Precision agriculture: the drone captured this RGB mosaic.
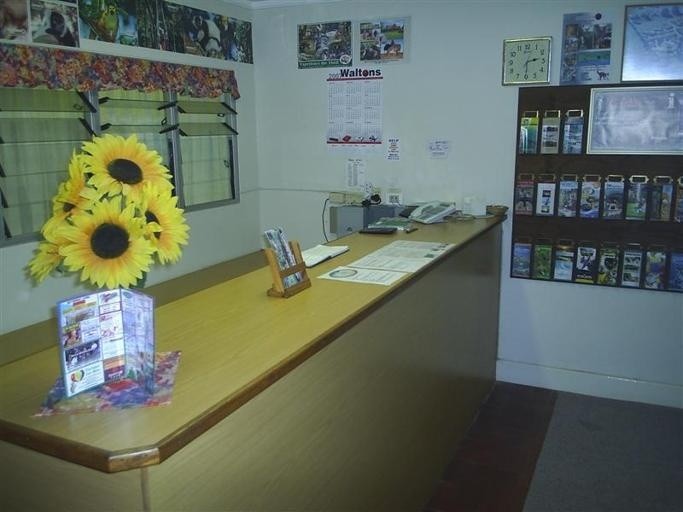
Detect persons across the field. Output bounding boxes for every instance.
[64,330,81,346]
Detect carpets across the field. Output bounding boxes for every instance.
[428,382,558,511]
[521,390,683,512]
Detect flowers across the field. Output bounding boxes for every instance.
[22,132,190,292]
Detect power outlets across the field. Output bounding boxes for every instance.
[329,192,345,203]
[389,195,399,203]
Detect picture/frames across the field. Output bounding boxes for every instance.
[586,87,683,156]
[620,2,683,84]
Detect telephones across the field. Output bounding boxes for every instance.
[408,201,457,225]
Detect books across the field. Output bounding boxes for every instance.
[367,217,409,230]
[301,242,349,268]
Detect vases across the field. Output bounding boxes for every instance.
[118,271,147,290]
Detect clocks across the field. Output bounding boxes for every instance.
[502,36,552,86]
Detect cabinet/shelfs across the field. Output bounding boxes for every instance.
[496,84,683,409]
